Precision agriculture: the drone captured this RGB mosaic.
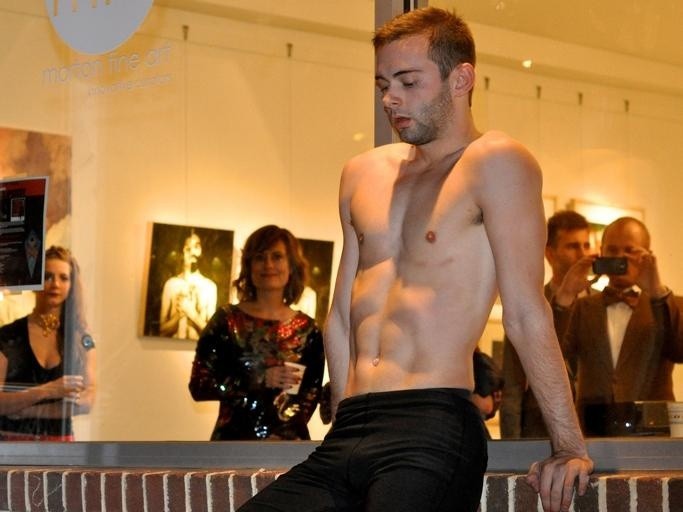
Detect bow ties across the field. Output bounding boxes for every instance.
[602,286,640,310]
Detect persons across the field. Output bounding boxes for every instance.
[158,233,218,341]
[468,348,504,419]
[230,4,594,512]
[0,246,95,441]
[549,216,683,434]
[285,284,318,320]
[500,210,604,440]
[187,224,326,442]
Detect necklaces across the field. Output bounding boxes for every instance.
[31,307,61,337]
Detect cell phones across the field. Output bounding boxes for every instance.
[593,257,627,274]
